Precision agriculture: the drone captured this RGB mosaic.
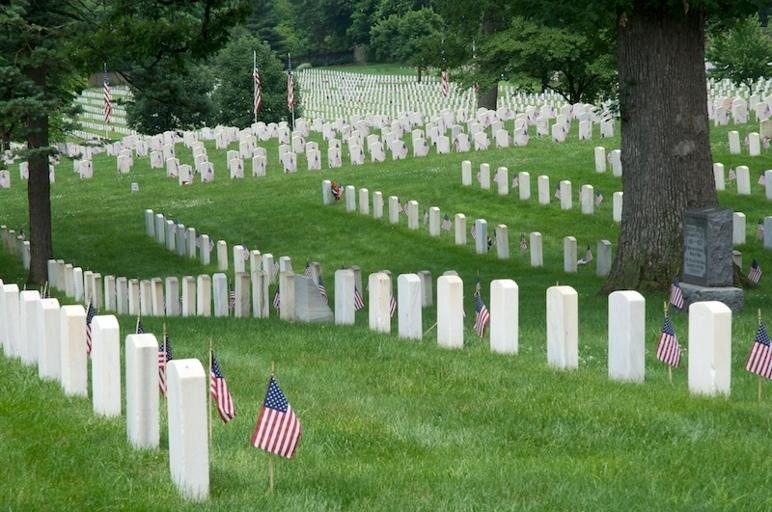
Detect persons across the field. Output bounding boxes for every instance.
[397,194,594,265]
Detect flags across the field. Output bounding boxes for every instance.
[438,40,451,97]
[207,346,238,424]
[21,280,174,401]
[655,258,772,381]
[250,51,263,115]
[224,253,491,341]
[248,374,303,460]
[101,60,115,126]
[727,127,772,251]
[282,50,298,116]
[475,169,604,208]
[331,185,344,200]
